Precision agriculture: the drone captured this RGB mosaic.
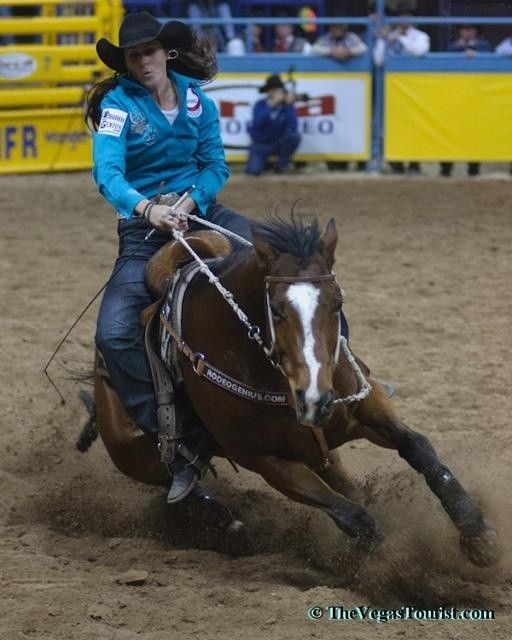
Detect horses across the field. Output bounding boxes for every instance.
[56,193,502,570]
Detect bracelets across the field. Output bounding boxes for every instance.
[146,202,156,225]
[139,201,153,220]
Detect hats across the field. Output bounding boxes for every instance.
[96,12,192,73]
[258,75,285,92]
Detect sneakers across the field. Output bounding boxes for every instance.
[376,384,393,399]
[168,447,211,503]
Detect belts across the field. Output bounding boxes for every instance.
[116,192,181,219]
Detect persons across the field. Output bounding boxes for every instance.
[244,9,512,178]
[84,11,275,503]
